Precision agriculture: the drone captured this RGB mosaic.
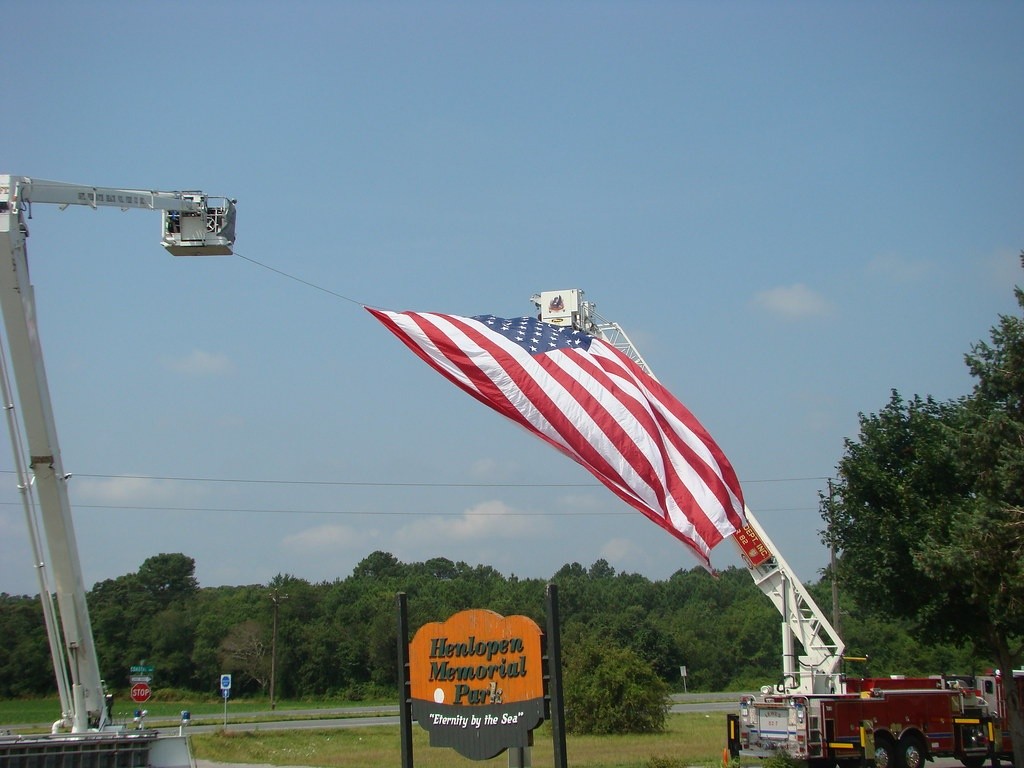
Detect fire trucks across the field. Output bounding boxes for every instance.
[527,287,1024,768]
[1,173,235,768]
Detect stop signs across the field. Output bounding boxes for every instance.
[130,683,151,703]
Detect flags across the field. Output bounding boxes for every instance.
[364,306,748,577]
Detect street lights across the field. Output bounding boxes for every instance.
[268,588,290,711]
